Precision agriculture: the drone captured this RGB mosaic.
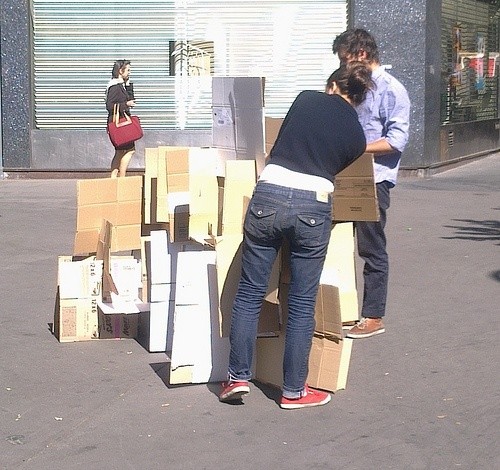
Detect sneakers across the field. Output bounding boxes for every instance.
[220,381,250,401]
[280,385,331,408]
[347,317,385,339]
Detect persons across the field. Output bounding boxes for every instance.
[104,60,136,179]
[220,61,375,410]
[333,29,410,339]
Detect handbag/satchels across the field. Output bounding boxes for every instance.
[108,103,143,148]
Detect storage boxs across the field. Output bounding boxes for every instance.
[54,77,380,392]
